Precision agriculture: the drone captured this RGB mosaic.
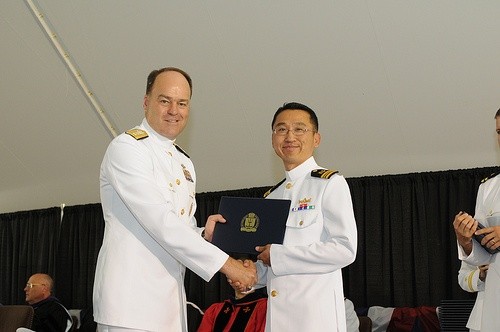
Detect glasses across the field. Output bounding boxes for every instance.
[272,126,316,136]
[26,282,49,288]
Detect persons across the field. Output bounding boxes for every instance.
[197,256,361,332]
[453,108,500,332]
[227,103,357,332]
[93,66,258,332]
[24,273,76,332]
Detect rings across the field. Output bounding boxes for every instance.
[247,284,252,291]
